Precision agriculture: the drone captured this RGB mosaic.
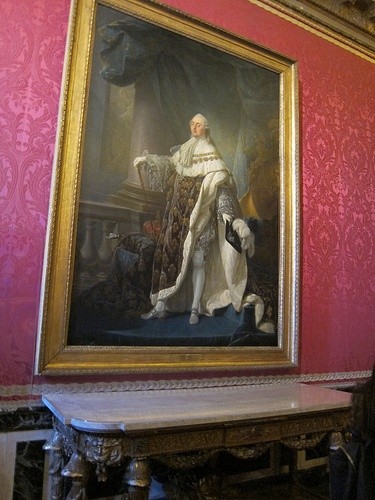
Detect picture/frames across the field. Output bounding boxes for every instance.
[33,0,301,375]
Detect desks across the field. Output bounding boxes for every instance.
[41,382,366,500]
